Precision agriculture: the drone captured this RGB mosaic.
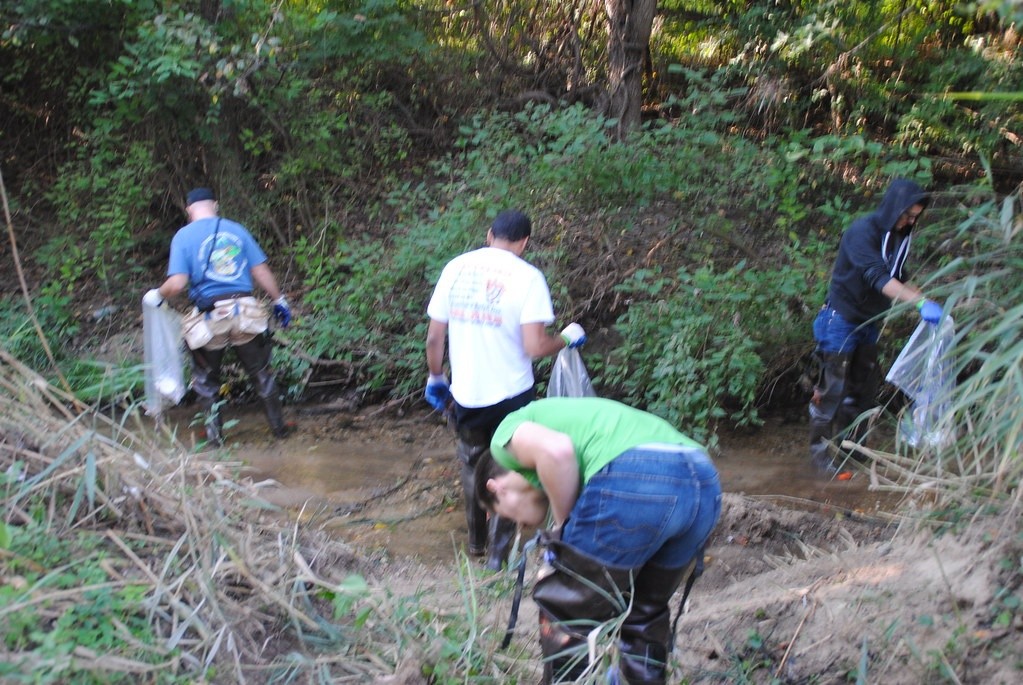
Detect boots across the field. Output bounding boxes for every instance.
[531,529,644,685]
[457,439,490,554]
[487,514,516,573]
[230,332,298,439]
[193,347,225,446]
[609,565,684,685]
[838,367,885,469]
[805,349,853,481]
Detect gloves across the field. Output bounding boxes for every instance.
[561,322,587,346]
[144,288,165,309]
[916,297,943,321]
[424,374,450,410]
[272,298,291,325]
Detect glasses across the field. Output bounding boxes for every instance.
[906,209,919,219]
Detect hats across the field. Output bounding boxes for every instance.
[186,188,215,204]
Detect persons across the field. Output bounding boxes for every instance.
[809,179,942,476]
[475,397,723,685]
[143,187,291,447]
[425,211,586,588]
